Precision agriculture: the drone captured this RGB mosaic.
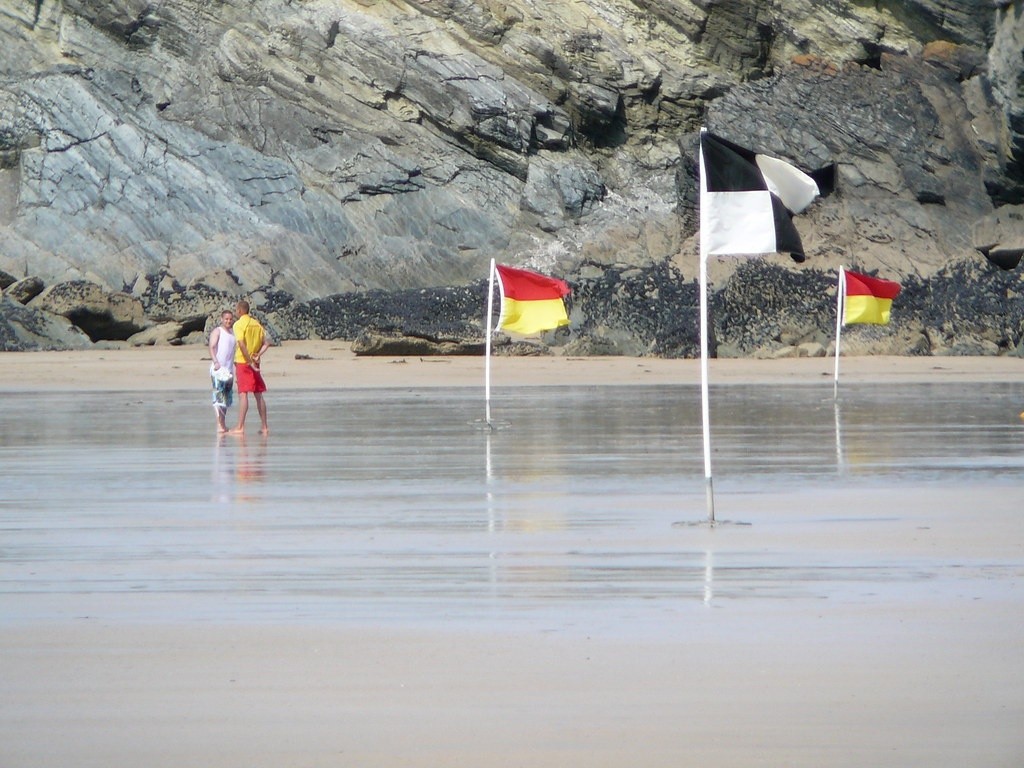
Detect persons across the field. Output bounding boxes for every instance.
[209,311,238,433]
[228,302,271,436]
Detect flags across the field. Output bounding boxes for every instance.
[494,265,573,339]
[702,129,820,261]
[840,267,902,327]
